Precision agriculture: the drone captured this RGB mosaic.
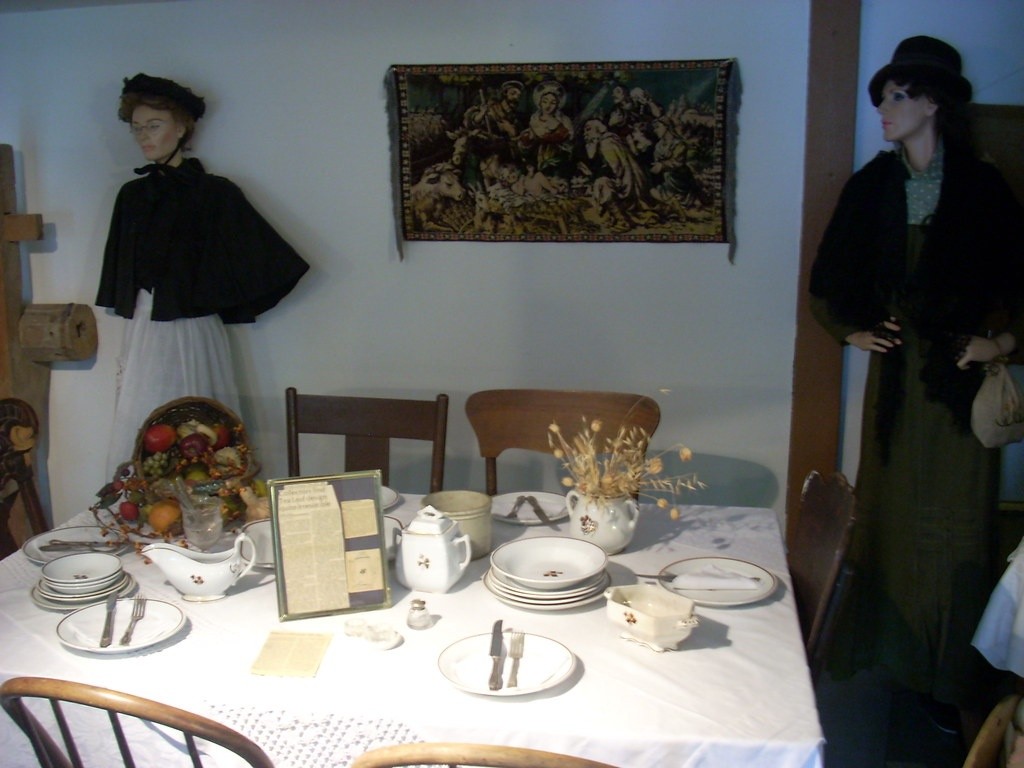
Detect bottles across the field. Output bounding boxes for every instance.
[407,600,432,630]
[391,506,471,593]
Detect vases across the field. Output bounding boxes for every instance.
[566,490,637,557]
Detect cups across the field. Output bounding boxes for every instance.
[181,496,223,551]
[420,489,493,561]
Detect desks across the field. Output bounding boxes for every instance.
[0,494,825,768]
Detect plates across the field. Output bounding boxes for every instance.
[381,485,398,510]
[32,552,135,610]
[438,632,576,696]
[484,537,615,611]
[656,557,777,606]
[23,524,130,563]
[492,491,569,524]
[238,518,276,569]
[55,597,186,654]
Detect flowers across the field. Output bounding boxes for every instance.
[547,385,708,521]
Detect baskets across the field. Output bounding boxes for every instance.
[132,396,252,490]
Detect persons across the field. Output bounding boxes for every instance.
[807,35,1024,733]
[95,73,311,483]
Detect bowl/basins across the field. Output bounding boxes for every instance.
[604,585,699,652]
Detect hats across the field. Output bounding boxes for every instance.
[868,34,973,116]
[124,72,206,122]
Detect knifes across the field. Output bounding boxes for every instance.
[488,620,503,690]
[630,572,675,582]
[100,590,118,647]
[526,496,560,531]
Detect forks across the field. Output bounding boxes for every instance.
[120,595,146,645]
[509,632,524,687]
[504,496,525,519]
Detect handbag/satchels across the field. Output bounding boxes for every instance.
[970,360,1024,448]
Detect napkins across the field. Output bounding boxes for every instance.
[670,565,763,590]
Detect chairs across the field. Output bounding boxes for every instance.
[0,388,862,768]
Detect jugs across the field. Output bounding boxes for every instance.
[141,534,257,601]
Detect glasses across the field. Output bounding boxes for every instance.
[127,118,178,137]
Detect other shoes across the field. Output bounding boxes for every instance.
[936,698,960,734]
